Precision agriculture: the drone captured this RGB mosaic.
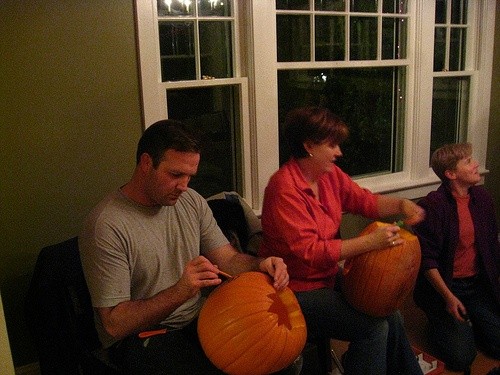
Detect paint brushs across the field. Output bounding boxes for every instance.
[218,270,233,279]
[458,308,472,327]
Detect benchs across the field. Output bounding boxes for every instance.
[27,191,330,375]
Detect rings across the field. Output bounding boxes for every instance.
[392,241,396,247]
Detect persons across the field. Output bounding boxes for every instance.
[259,107,428,375]
[410,143,500,373]
[77,118,307,375]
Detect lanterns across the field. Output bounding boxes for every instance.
[196,272,307,375]
[340,221,422,319]
